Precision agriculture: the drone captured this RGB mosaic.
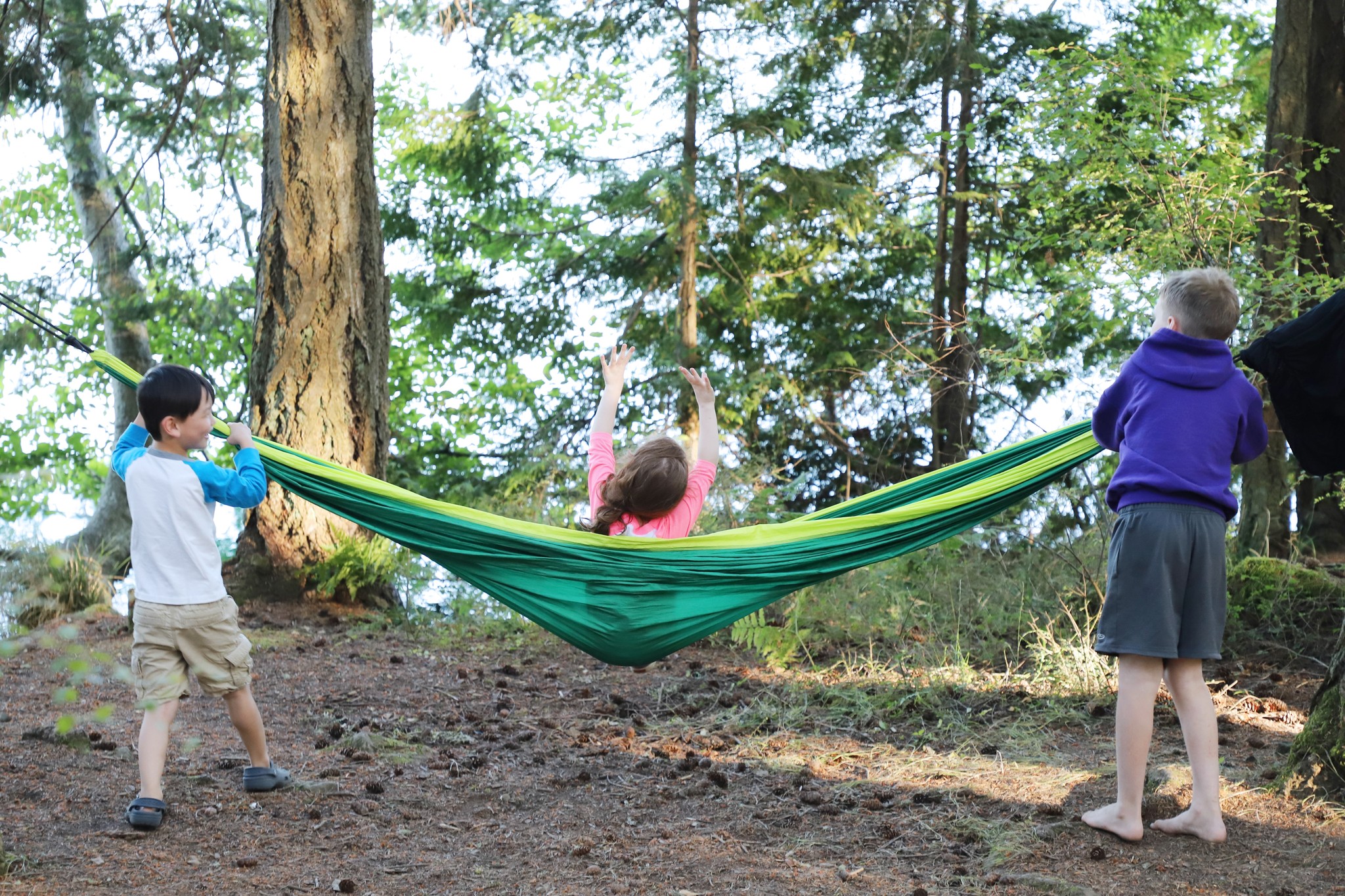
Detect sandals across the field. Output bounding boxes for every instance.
[242,763,292,790]
[125,798,165,827]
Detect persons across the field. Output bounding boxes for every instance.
[580,344,720,672]
[1080,265,1270,840]
[110,364,292,827]
